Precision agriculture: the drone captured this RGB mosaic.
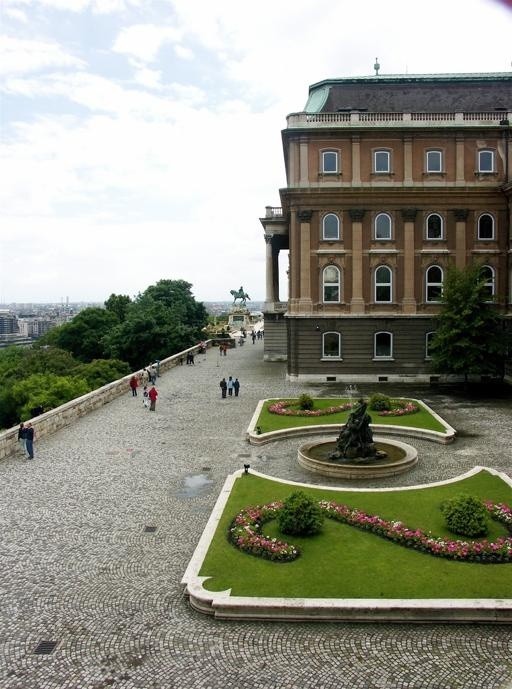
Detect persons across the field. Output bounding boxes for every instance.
[186,351,190,364]
[219,378,227,398]
[149,386,158,411]
[228,376,234,397]
[24,423,34,460]
[136,362,158,387]
[130,376,138,397]
[238,285,243,294]
[234,378,240,397]
[243,329,264,344]
[224,344,226,356]
[219,345,224,356]
[335,398,372,459]
[18,422,28,455]
[143,386,149,408]
[190,352,194,364]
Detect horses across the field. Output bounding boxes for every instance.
[230,290,250,304]
